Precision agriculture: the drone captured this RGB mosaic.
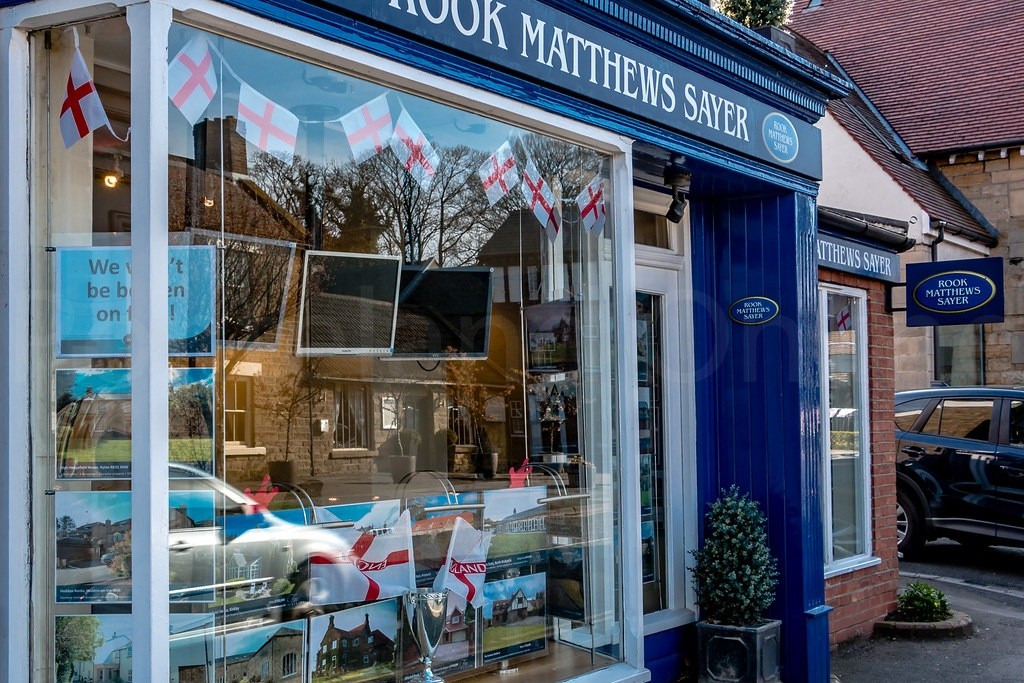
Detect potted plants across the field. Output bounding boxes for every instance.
[686,484,782,683]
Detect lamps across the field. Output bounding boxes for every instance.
[205,177,217,207]
[105,156,125,187]
[663,163,691,224]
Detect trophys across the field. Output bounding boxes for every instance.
[402,587,448,682]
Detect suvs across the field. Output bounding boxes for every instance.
[895,385,1024,565]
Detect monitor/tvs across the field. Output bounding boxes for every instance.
[184,225,494,362]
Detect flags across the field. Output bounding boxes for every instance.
[310,508,417,606]
[58,48,108,149]
[440,516,492,610]
[574,173,606,237]
[235,82,300,167]
[339,93,440,191]
[478,141,561,244]
[167,32,218,128]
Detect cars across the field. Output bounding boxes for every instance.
[53,460,361,680]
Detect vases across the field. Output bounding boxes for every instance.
[266,460,297,492]
[471,452,499,480]
[388,455,416,485]
[563,462,595,488]
